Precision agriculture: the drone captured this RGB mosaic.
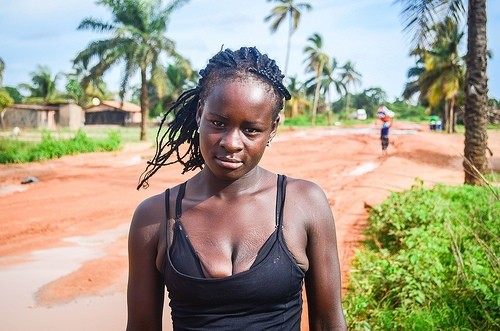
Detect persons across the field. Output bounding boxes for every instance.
[126,46,347,330]
[380,118,392,149]
